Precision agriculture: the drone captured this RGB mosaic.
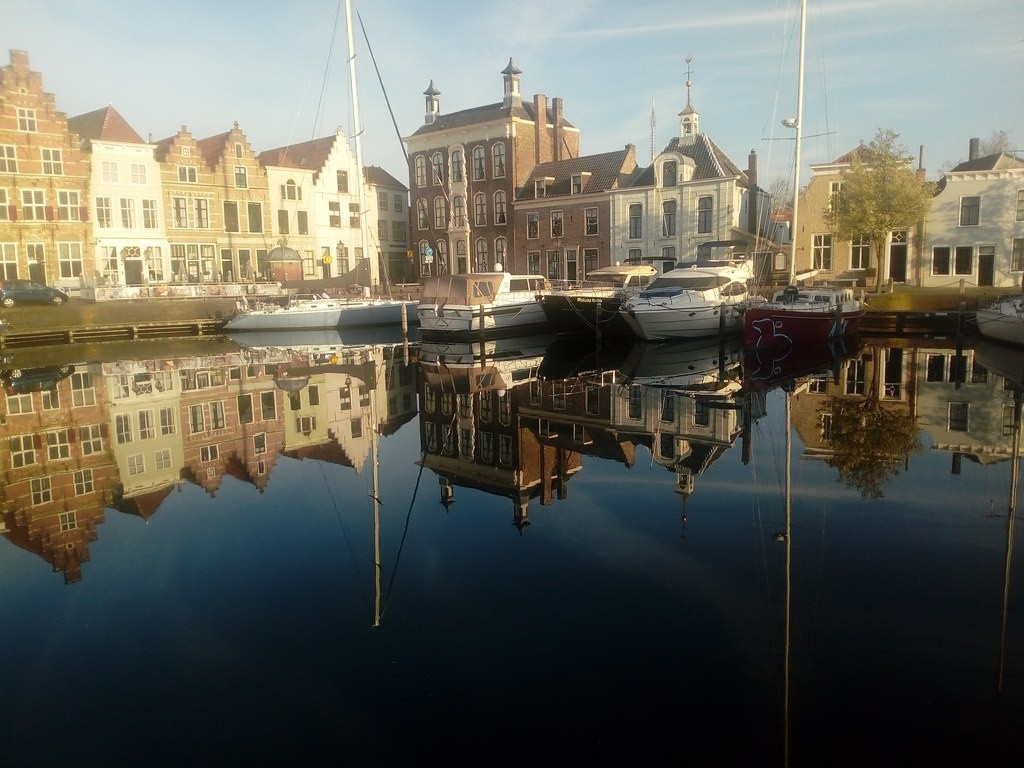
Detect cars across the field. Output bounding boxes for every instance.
[0,280,70,308]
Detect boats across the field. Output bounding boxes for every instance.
[616,340,745,399]
[619,259,748,339]
[416,273,551,340]
[412,341,548,397]
[225,332,437,629]
[536,266,656,331]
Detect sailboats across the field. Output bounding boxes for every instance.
[976,293,1024,345]
[972,346,1023,695]
[225,1,419,332]
[743,1,862,342]
[741,341,857,767]
